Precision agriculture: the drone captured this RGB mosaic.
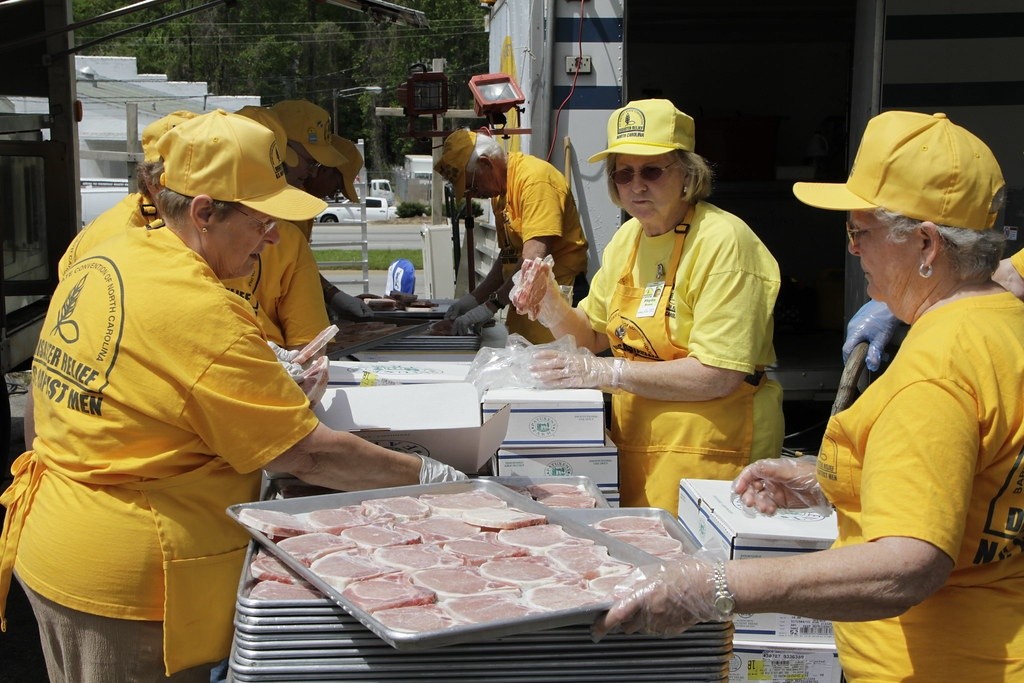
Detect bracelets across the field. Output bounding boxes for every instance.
[611,358,625,392]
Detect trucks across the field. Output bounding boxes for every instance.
[336,178,395,207]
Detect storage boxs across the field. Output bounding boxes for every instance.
[264,383,620,509]
[698,116,791,180]
[677,478,842,683]
[816,267,844,330]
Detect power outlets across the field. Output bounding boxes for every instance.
[566,56,592,74]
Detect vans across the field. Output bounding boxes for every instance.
[315,196,388,225]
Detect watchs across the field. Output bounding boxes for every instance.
[489,292,505,310]
[713,561,735,618]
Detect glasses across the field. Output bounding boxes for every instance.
[287,143,322,170]
[462,155,488,197]
[215,200,278,235]
[846,219,956,249]
[609,157,680,185]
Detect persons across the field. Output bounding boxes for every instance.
[9,110,470,683]
[842,244,1024,372]
[58,97,374,385]
[590,109,1024,683]
[514,97,785,520]
[431,129,591,347]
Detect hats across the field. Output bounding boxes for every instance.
[156,109,328,220]
[434,129,477,205]
[323,135,364,204]
[234,105,299,167]
[273,99,349,167]
[792,111,1007,232]
[141,110,201,165]
[587,99,695,164]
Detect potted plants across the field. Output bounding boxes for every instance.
[444,198,484,225]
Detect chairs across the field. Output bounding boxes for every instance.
[353,259,416,299]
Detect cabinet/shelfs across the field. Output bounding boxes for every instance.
[420,223,479,299]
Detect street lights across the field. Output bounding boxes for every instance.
[332,85,381,205]
